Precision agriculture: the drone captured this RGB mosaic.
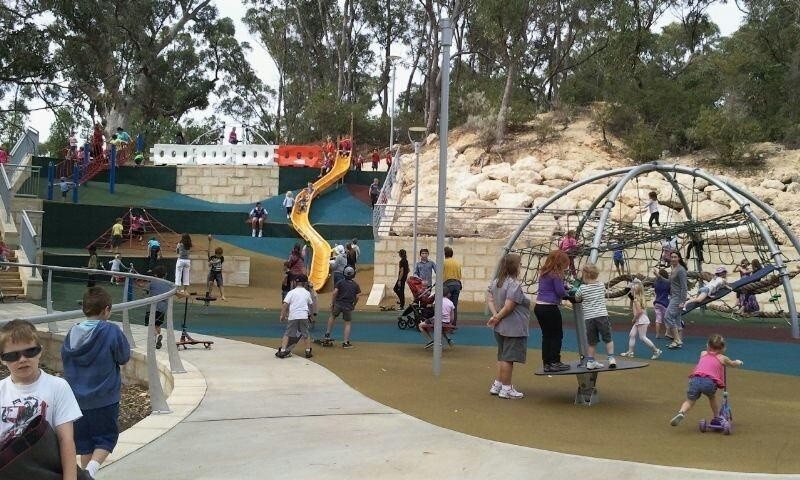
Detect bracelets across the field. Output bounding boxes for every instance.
[497,312,504,320]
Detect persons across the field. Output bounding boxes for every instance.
[0,319,83,479]
[393,248,410,310]
[54,176,74,201]
[60,285,131,479]
[737,259,762,314]
[442,246,463,328]
[418,286,455,350]
[323,266,362,348]
[558,230,580,276]
[614,240,625,276]
[85,212,163,294]
[575,262,617,370]
[207,247,226,301]
[228,127,239,145]
[143,264,191,349]
[670,334,743,429]
[686,231,705,262]
[0,147,8,163]
[277,275,314,359]
[417,281,431,299]
[664,250,688,350]
[484,253,530,399]
[653,269,673,339]
[644,191,661,229]
[619,283,663,361]
[62,123,146,190]
[0,231,11,271]
[734,259,751,306]
[533,250,575,373]
[250,202,269,238]
[281,132,395,286]
[174,233,193,295]
[683,267,733,309]
[413,248,437,296]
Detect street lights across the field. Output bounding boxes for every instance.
[413,141,423,276]
[434,19,456,372]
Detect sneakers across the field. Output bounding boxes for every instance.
[343,340,354,348]
[281,349,289,355]
[489,334,684,427]
[325,333,330,339]
[424,341,434,349]
[305,347,312,358]
[156,334,163,349]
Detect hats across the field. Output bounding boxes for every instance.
[343,266,356,280]
[295,274,309,282]
[715,266,727,276]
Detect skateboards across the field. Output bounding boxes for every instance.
[275,329,301,358]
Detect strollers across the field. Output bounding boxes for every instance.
[397,275,436,329]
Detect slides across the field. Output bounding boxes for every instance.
[291,149,352,291]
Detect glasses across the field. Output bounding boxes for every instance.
[0,346,41,363]
